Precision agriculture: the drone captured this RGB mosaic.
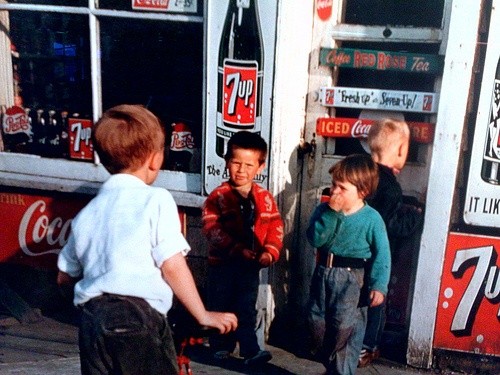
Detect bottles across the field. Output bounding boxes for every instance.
[72,112,80,118]
[0,103,7,152]
[480,56,500,186]
[215,0,265,159]
[24,107,32,114]
[36,108,69,158]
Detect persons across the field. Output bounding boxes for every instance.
[357,118,409,367]
[202,131,283,368]
[308,154,391,375]
[57,105,237,375]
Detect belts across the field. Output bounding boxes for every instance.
[316,252,371,269]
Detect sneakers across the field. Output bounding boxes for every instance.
[357,348,380,367]
[214,350,229,359]
[244,350,272,367]
